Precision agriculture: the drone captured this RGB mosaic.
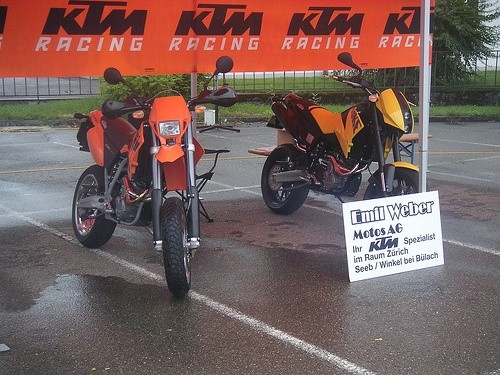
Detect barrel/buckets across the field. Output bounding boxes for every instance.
[205,109,215,127]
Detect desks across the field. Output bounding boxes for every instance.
[247,132,437,165]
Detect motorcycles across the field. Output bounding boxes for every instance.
[261,51,431,215]
[71,55,241,296]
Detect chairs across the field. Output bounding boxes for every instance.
[195,148,231,223]
